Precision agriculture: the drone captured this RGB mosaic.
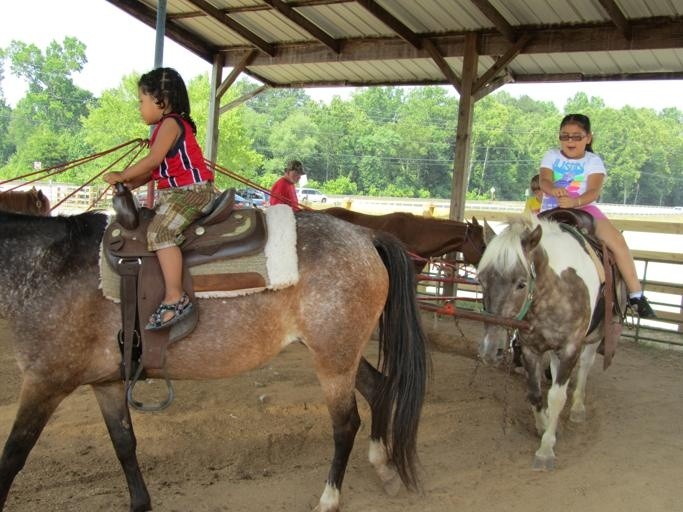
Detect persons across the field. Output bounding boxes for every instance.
[100,67,214,331]
[539,113,656,319]
[523,173,545,215]
[268,159,306,212]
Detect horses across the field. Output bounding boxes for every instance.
[0,184,51,217]
[476,214,626,471]
[0,207,431,512]
[313,206,487,290]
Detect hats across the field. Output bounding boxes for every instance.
[285,160,306,175]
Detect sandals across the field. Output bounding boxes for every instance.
[145,293,192,331]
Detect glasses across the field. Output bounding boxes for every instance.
[559,135,584,141]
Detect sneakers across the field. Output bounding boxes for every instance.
[630,297,656,319]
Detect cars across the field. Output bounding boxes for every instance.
[294,187,327,203]
[234,187,270,208]
